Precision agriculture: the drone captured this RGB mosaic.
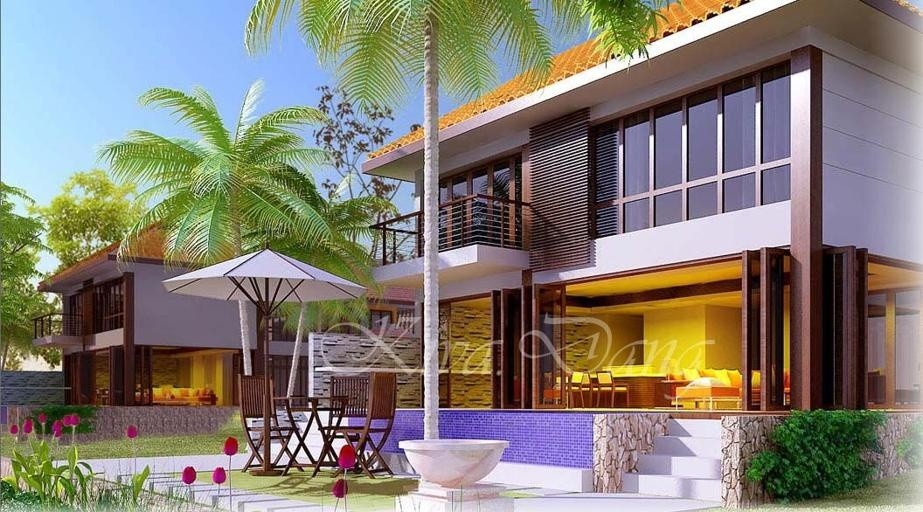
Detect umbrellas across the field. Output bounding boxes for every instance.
[159,240,368,476]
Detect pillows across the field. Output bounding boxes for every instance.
[682,367,760,387]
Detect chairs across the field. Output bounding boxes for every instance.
[237,370,398,480]
[555,370,630,408]
